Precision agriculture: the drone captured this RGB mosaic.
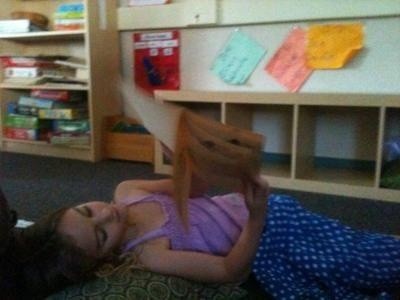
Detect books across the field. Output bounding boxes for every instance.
[0,3,85,34]
[0,53,89,86]
[113,74,266,237]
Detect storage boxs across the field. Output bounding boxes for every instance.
[101,113,154,163]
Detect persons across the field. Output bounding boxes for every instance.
[25,168,400,299]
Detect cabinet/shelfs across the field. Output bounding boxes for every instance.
[154,90,399,202]
[0,0,124,163]
